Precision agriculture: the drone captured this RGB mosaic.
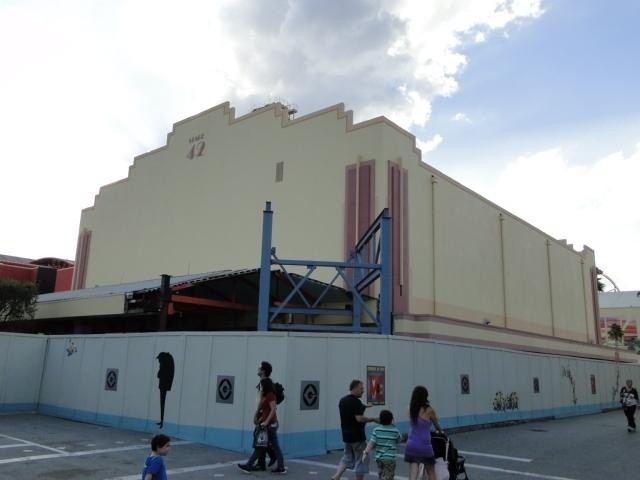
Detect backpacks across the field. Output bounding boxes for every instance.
[272,381,285,405]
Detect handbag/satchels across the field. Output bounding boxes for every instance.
[254,428,268,447]
[267,420,279,432]
[417,436,450,480]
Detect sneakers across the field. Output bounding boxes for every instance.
[236,454,287,475]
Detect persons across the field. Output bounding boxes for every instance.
[141,434,171,480]
[237,361,287,474]
[330,380,442,480]
[619,379,638,432]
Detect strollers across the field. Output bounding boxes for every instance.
[422,429,470,480]
